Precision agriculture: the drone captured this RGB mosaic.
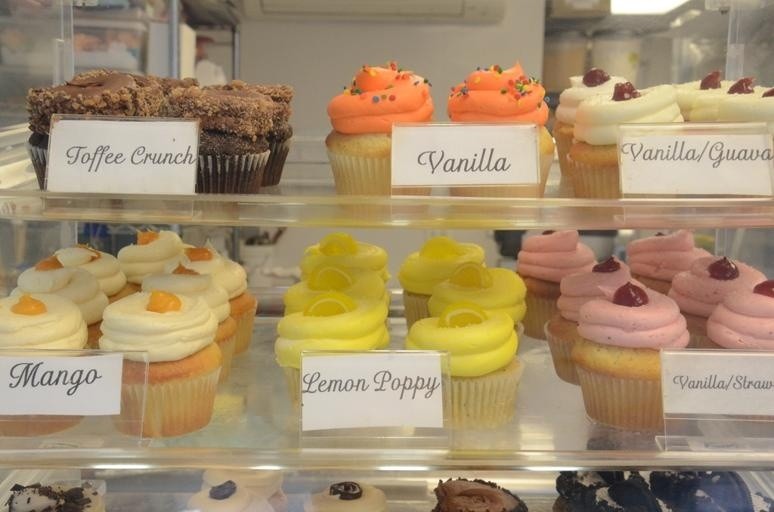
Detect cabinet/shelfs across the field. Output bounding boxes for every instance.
[0,137,774,512]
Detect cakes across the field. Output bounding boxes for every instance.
[397,236,485,336]
[430,477,528,512]
[0,292,89,355]
[300,233,392,284]
[186,480,275,512]
[324,61,430,198]
[514,229,599,340]
[705,280,774,353]
[717,86,774,125]
[140,261,236,383]
[673,69,735,121]
[0,439,107,512]
[627,228,711,296]
[274,291,388,410]
[554,67,627,174]
[428,262,528,355]
[571,282,691,432]
[98,291,222,437]
[26,71,198,195]
[543,255,632,387]
[446,61,555,199]
[10,255,109,350]
[164,237,257,354]
[220,79,294,186]
[157,86,274,196]
[690,75,768,123]
[116,227,183,293]
[201,453,288,512]
[406,302,525,421]
[283,262,388,320]
[667,255,766,350]
[565,81,685,198]
[52,245,135,304]
[304,479,387,512]
